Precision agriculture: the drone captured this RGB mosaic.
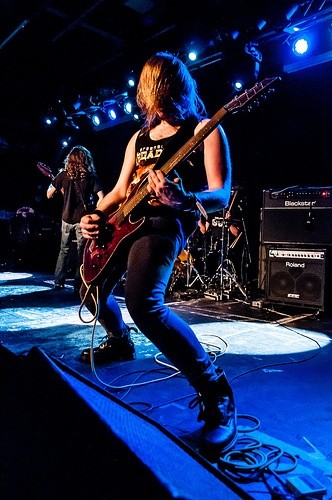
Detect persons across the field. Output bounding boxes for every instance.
[46,145,104,290]
[186,186,248,294]
[77,54,238,456]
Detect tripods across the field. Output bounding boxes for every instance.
[169,209,248,303]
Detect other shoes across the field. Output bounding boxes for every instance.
[55,284,64,289]
[75,286,79,290]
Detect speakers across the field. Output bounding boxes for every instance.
[0,347,257,500]
[264,245,332,311]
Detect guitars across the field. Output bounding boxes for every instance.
[37,162,64,196]
[82,77,283,286]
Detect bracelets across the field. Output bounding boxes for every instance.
[188,191,208,220]
[89,210,107,222]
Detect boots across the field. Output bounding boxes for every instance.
[188,369,237,457]
[83,334,134,364]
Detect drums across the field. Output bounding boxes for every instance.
[198,185,244,222]
[174,250,194,278]
